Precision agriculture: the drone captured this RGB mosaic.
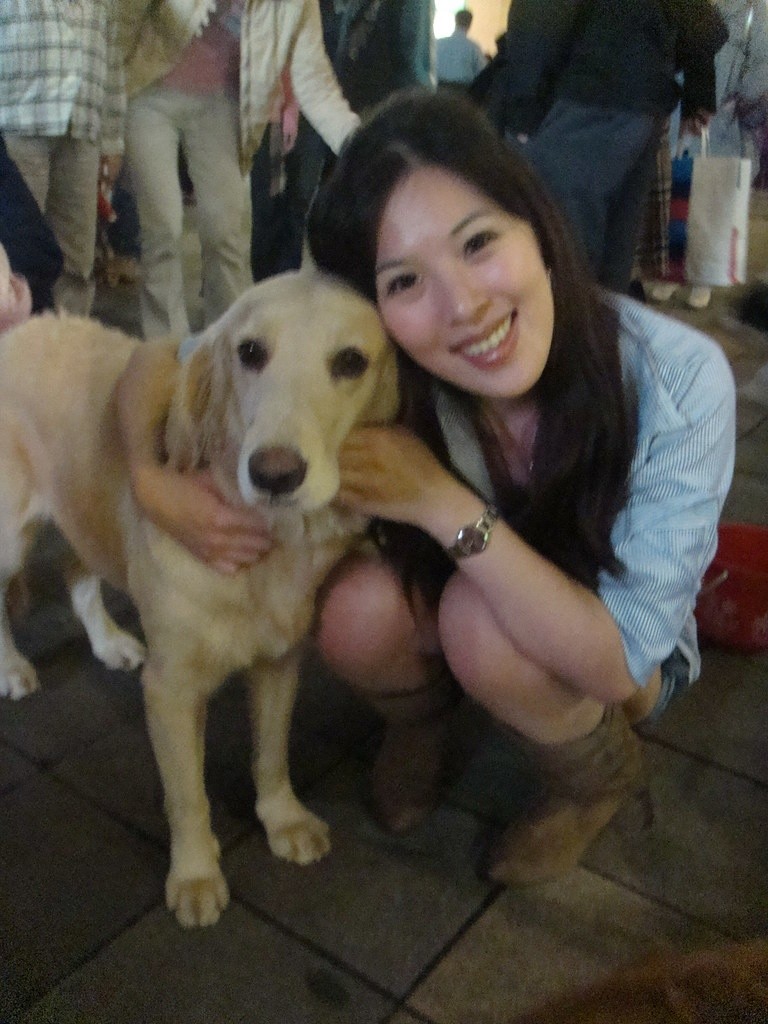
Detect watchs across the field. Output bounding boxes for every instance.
[444,505,500,560]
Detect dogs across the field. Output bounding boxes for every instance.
[0,274,398,936]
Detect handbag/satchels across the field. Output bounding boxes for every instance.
[670,124,750,286]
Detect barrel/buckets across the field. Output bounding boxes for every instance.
[697,521,768,651]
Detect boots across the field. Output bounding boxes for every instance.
[358,681,456,834]
[484,704,654,891]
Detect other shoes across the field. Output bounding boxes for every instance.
[651,279,678,303]
[688,284,715,310]
[107,253,134,283]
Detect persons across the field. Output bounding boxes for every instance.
[429,9,488,93]
[463,0,768,310]
[114,90,735,883]
[250,0,431,284]
[0,0,126,331]
[106,0,361,341]
